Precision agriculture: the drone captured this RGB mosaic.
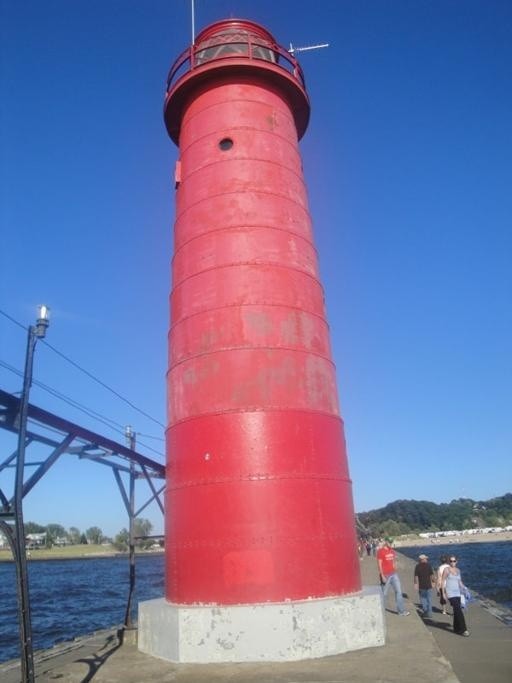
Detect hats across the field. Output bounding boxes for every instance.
[384,535,394,542]
[418,554,429,560]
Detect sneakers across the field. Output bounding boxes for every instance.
[398,610,410,616]
[459,630,470,636]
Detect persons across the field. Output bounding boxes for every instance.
[413,553,437,619]
[440,555,472,638]
[434,554,452,614]
[377,535,411,617]
[357,535,384,561]
